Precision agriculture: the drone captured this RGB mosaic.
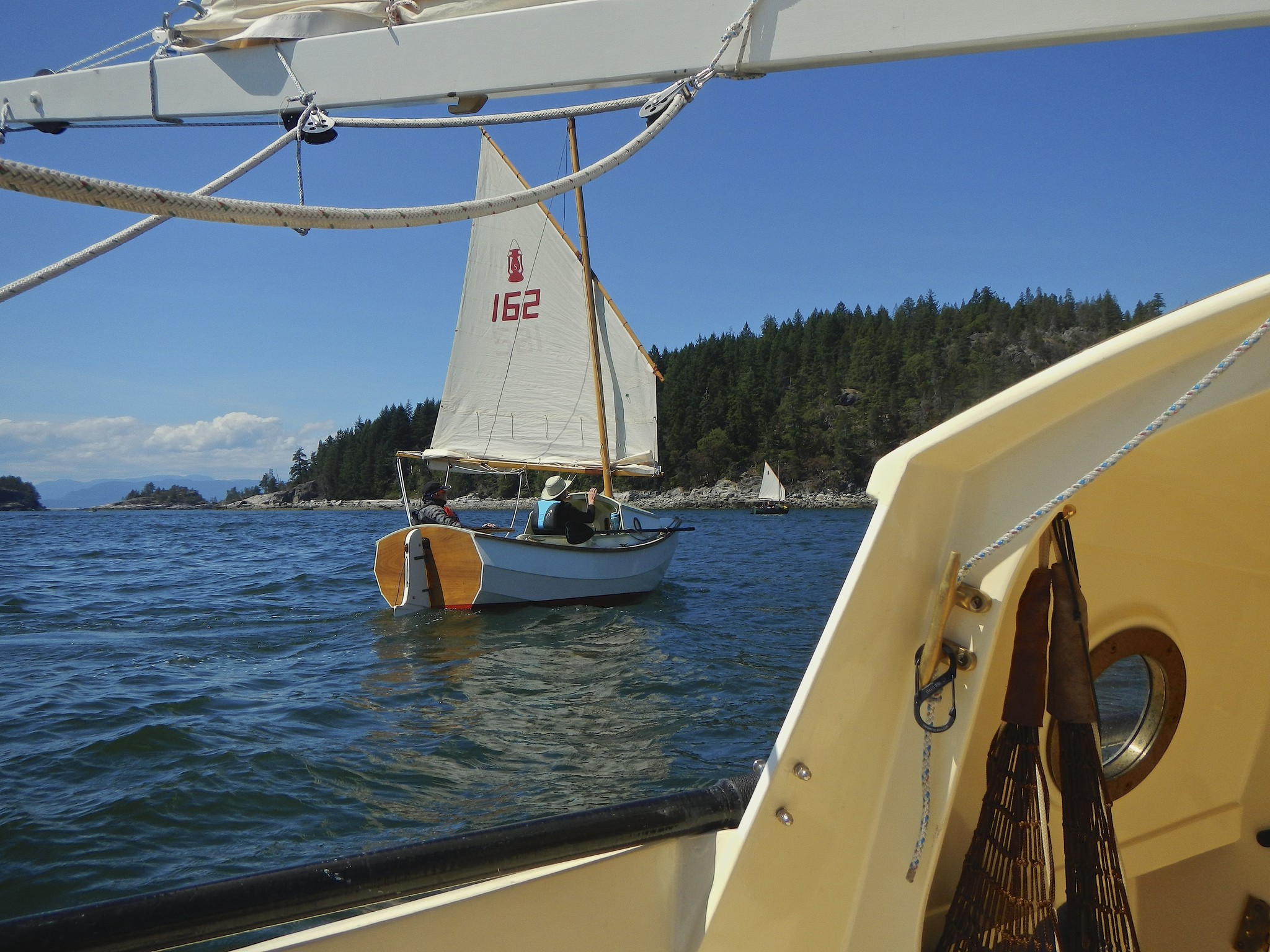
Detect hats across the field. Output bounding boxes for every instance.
[423,481,452,496]
[541,475,572,501]
[767,501,770,504]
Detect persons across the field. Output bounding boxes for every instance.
[531,475,597,537]
[417,481,496,529]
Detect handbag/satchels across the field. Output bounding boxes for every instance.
[934,509,1140,951]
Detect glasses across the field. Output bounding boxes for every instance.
[439,490,446,493]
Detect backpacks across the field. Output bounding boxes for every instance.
[531,500,562,534]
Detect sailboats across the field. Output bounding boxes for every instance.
[375,126,680,611]
[750,459,789,516]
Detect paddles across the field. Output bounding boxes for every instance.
[564,519,696,544]
